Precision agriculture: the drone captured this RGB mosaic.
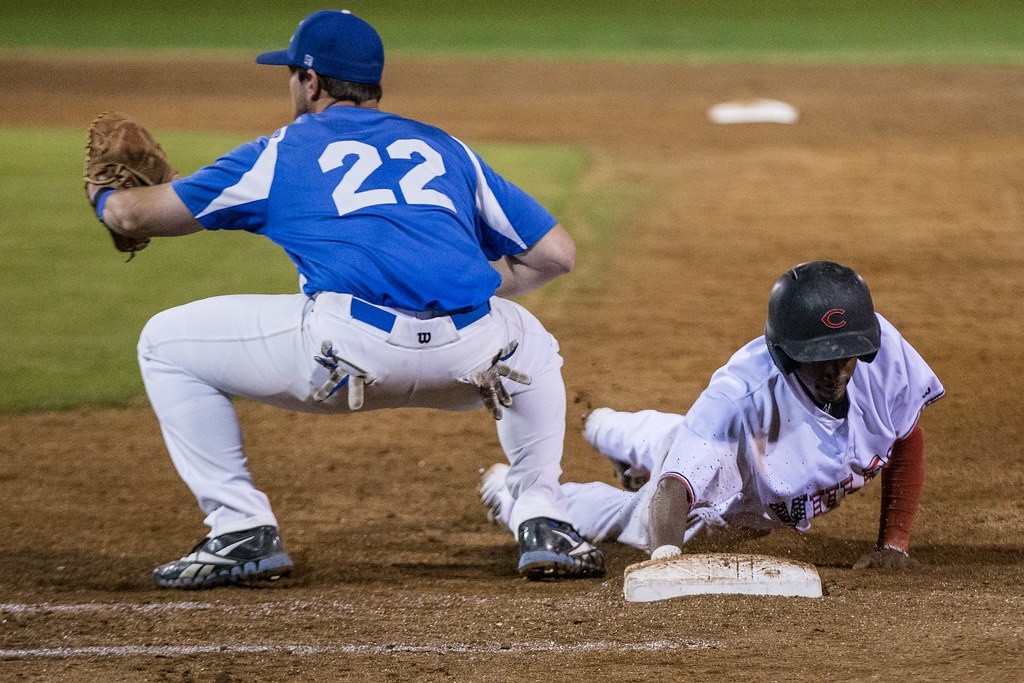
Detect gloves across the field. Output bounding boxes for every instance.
[854,546,919,571]
[314,342,364,410]
[472,340,531,420]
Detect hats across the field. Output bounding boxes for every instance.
[256,12,385,81]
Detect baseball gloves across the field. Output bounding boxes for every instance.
[84,110,181,253]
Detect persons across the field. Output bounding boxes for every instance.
[83,10,607,587]
[475,262,946,569]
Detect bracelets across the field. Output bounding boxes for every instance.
[96,190,115,219]
[651,545,682,560]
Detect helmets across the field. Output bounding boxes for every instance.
[765,261,882,377]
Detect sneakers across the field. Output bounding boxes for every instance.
[518,518,605,575]
[477,464,514,528]
[578,407,650,491]
[153,526,292,587]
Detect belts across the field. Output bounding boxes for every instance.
[351,299,489,333]
